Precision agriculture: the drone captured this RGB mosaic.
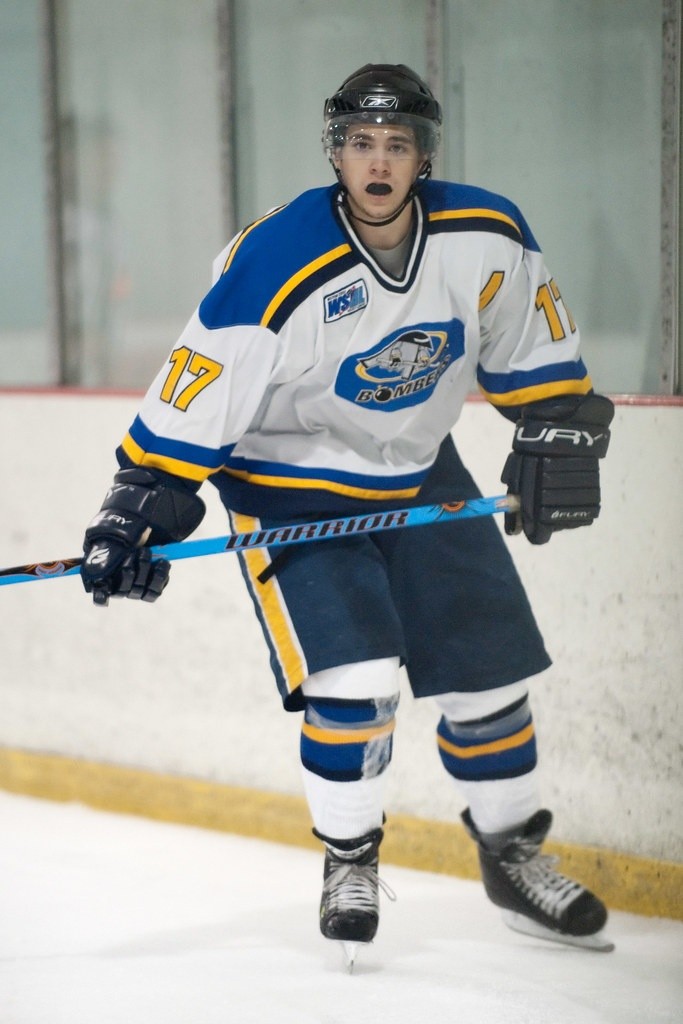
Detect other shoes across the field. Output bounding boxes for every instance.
[460,806,615,952]
[311,810,396,975]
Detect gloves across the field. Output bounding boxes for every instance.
[82,469,206,604]
[500,394,615,546]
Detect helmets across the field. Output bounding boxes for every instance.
[325,64,441,148]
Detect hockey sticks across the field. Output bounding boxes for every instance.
[0,495,520,583]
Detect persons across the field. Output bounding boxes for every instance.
[85,64,617,955]
